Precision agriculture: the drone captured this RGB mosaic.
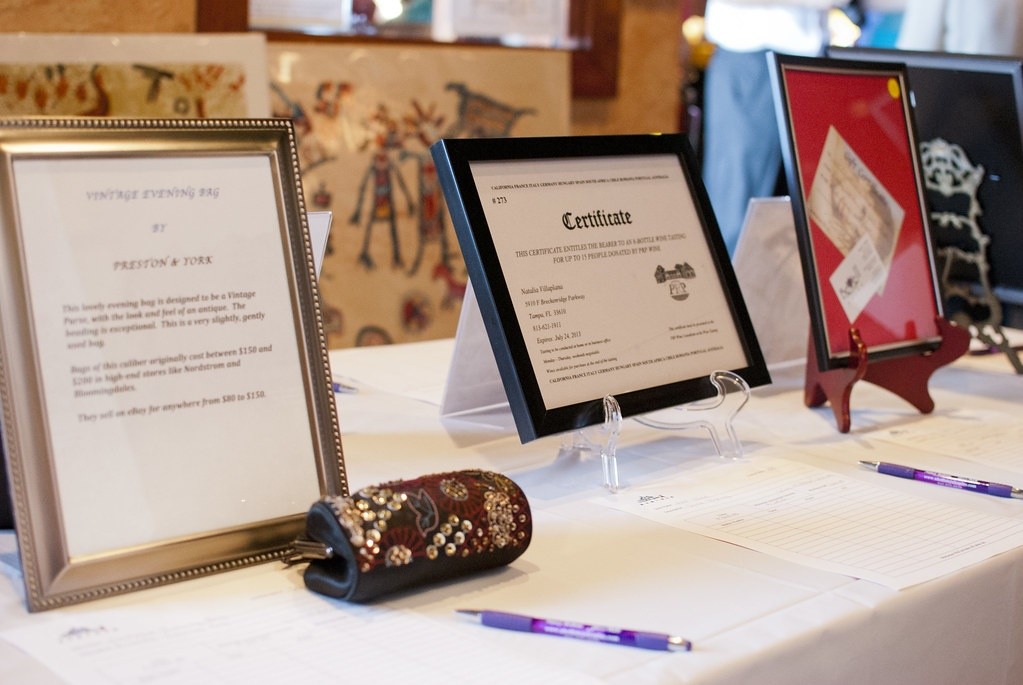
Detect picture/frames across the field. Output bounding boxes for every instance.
[765,49,943,371]
[1,118,350,613]
[430,129,775,445]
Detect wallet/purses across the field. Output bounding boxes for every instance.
[279,468,533,604]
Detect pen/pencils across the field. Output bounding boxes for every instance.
[453,608,694,653]
[856,459,1023,500]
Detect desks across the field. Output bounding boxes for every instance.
[0,323,1023,685]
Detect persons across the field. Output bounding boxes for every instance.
[703,0,855,252]
